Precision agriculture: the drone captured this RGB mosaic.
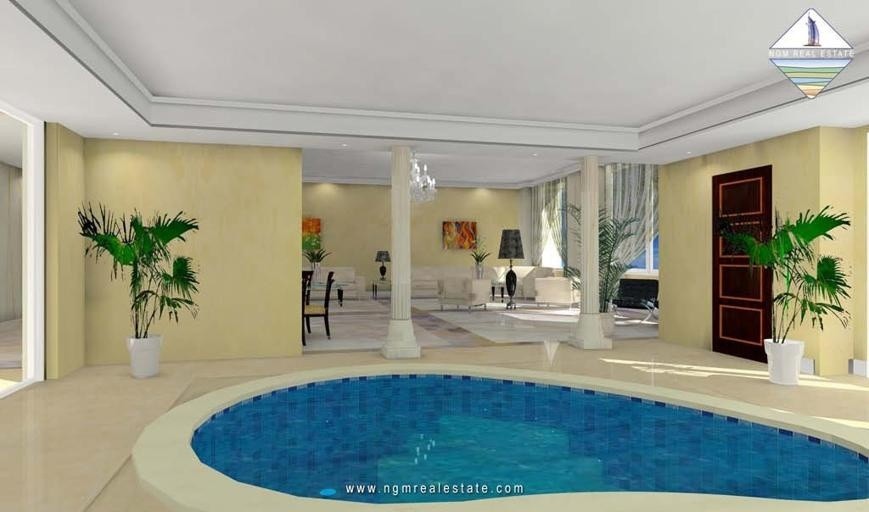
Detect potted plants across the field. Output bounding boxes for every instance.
[469,250,492,279]
[76,200,202,379]
[722,206,854,388]
[559,203,641,338]
[303,248,333,276]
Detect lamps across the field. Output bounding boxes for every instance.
[497,229,526,310]
[404,148,440,203]
[375,249,391,281]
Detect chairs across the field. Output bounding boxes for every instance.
[302,268,335,347]
